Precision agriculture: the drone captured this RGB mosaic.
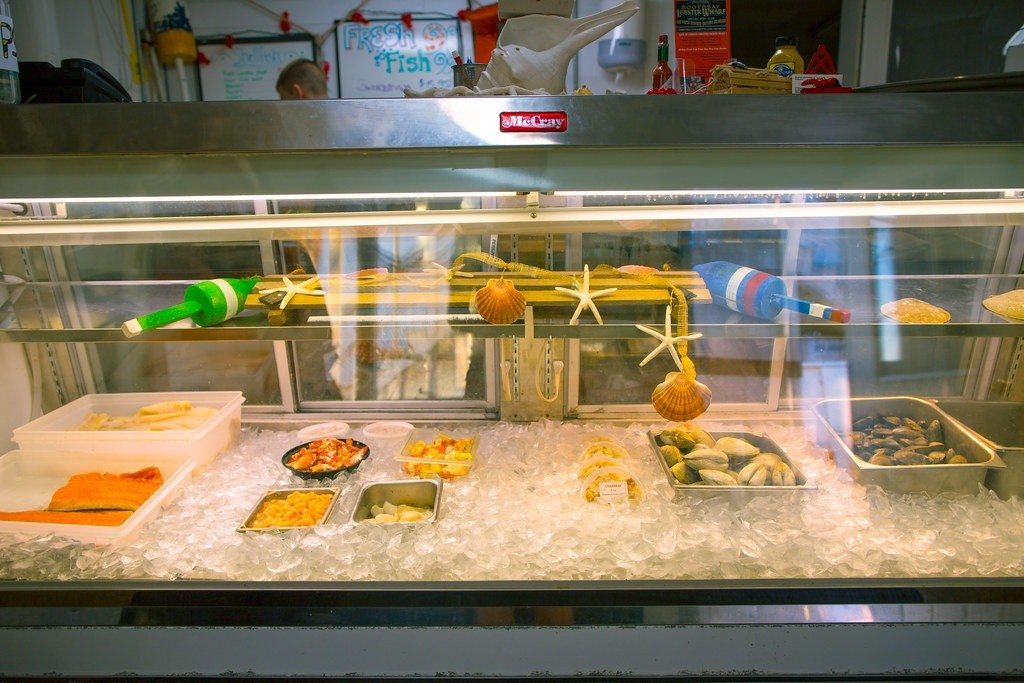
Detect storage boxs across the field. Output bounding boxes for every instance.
[0,391,247,545]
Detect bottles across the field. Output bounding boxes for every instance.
[767,35,805,75]
[651,35,673,92]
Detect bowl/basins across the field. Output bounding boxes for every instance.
[572,441,645,507]
[10,391,246,471]
[394,427,481,482]
[0,448,199,546]
[281,438,371,482]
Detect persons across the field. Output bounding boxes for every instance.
[274,57,329,100]
